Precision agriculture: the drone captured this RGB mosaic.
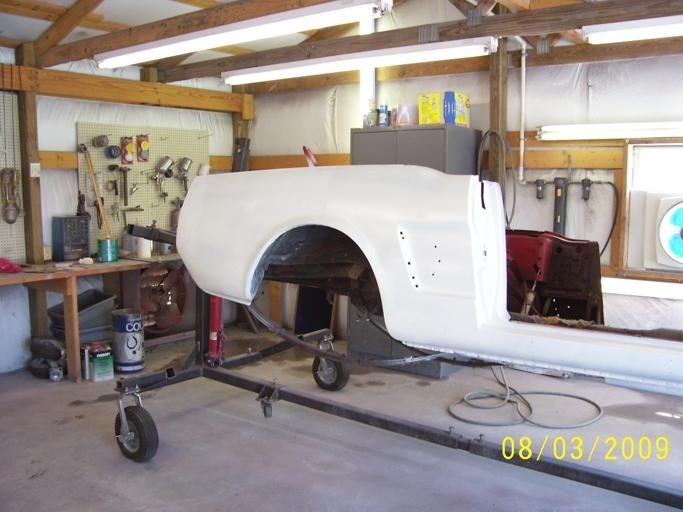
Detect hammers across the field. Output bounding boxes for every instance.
[109,165,131,206]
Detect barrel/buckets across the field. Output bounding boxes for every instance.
[97,239,118,263]
[112,309,145,375]
[80,342,114,381]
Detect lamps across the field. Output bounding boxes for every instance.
[535,121,683,142]
[93,0,393,70]
[582,11,682,46]
[221,36,499,86]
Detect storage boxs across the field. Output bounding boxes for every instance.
[419,91,471,128]
[47,289,117,343]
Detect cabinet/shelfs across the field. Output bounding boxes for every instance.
[346,124,483,380]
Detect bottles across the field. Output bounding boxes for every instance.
[378,99,389,129]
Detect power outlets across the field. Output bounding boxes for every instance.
[30,164,41,178]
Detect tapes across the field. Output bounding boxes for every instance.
[93,135,122,159]
